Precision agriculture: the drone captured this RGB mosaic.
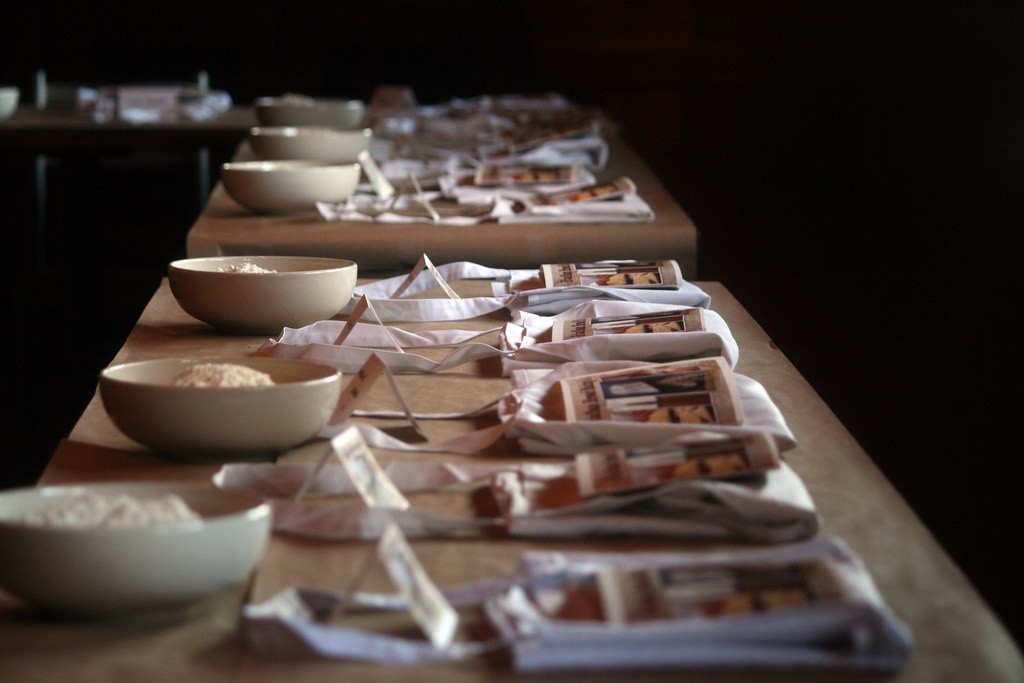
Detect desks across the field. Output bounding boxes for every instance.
[1,102,1024,683]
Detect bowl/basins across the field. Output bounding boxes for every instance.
[169,254,358,335]
[254,95,366,130]
[100,357,342,461]
[248,124,372,168]
[0,482,272,624]
[221,160,361,216]
